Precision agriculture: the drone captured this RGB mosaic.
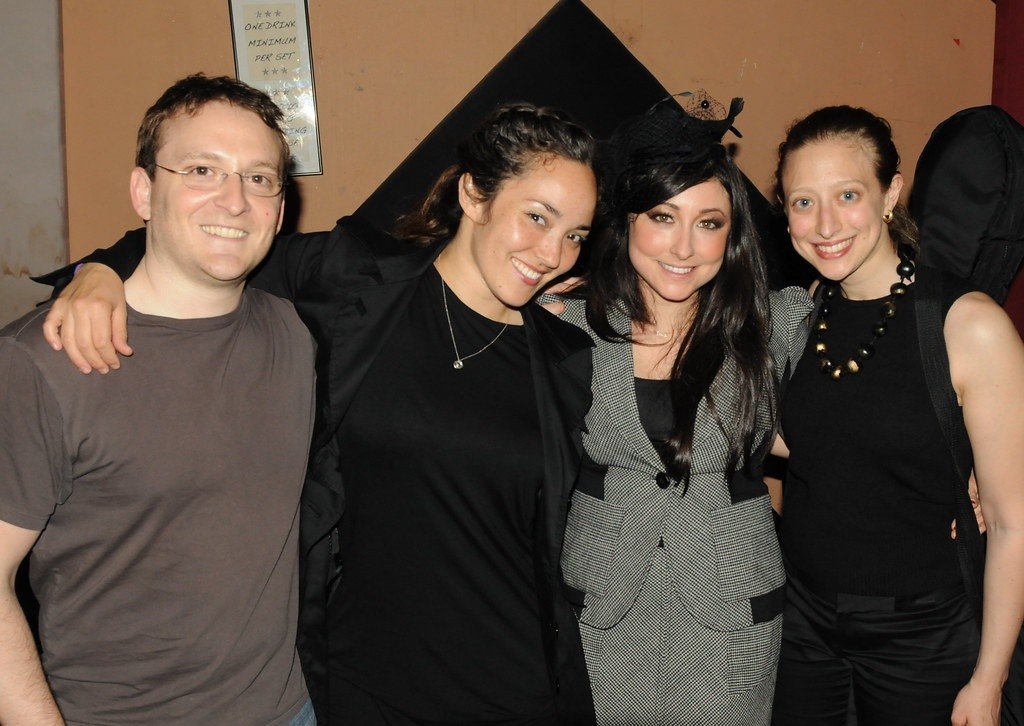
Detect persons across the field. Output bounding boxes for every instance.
[532,105,1024,726]
[0,70,598,726]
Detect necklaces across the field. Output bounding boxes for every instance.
[650,324,669,338]
[814,245,915,380]
[438,253,512,368]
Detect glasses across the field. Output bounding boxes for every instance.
[149,164,289,197]
